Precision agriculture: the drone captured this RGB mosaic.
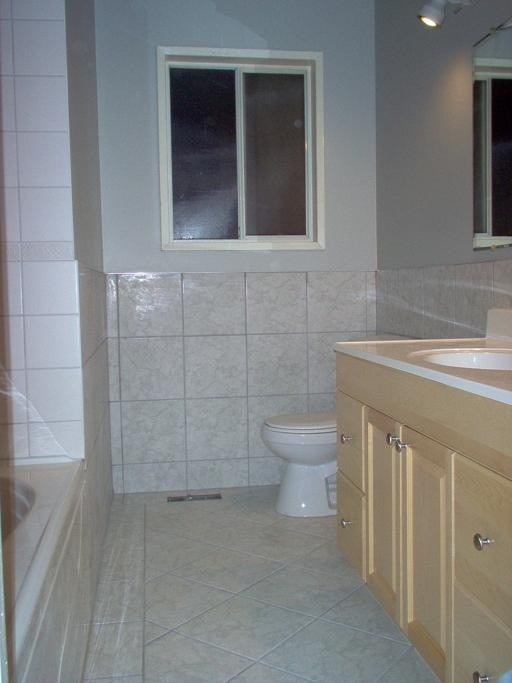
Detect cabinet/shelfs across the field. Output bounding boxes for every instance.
[337,390,368,584]
[453,452,512,682]
[367,409,454,682]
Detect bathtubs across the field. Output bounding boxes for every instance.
[0,460,87,682]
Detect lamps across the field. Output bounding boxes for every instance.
[416,0,469,29]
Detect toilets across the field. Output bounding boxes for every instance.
[258,413,340,518]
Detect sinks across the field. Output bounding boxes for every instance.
[405,348,512,373]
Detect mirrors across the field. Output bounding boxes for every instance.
[471,16,512,251]
